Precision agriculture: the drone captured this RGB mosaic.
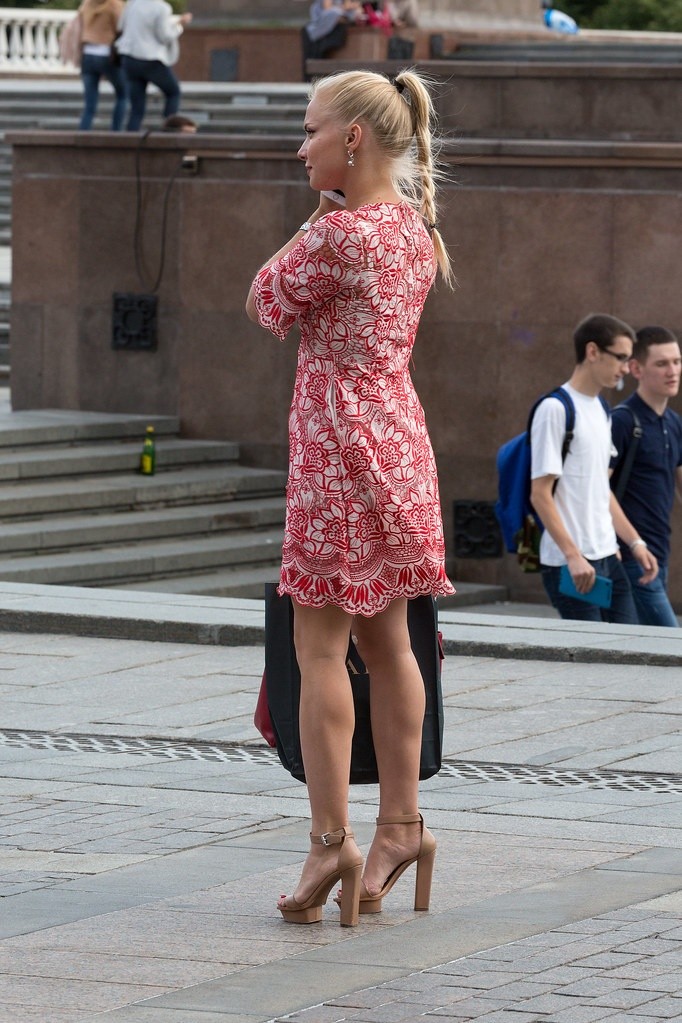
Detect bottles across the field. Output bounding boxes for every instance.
[141,426,156,475]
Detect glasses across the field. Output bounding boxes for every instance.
[601,347,631,363]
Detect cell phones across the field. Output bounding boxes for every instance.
[321,188,346,207]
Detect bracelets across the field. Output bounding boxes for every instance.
[300,222,313,234]
[628,538,648,551]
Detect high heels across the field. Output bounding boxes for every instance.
[277,826,363,928]
[333,813,436,911]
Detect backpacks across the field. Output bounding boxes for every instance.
[495,385,609,573]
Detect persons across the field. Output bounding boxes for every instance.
[73,0,196,132]
[530,313,682,627]
[301,0,412,79]
[245,68,457,928]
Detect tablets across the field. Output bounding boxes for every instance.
[557,565,612,607]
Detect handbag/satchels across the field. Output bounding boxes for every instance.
[58,12,81,67]
[254,583,445,785]
